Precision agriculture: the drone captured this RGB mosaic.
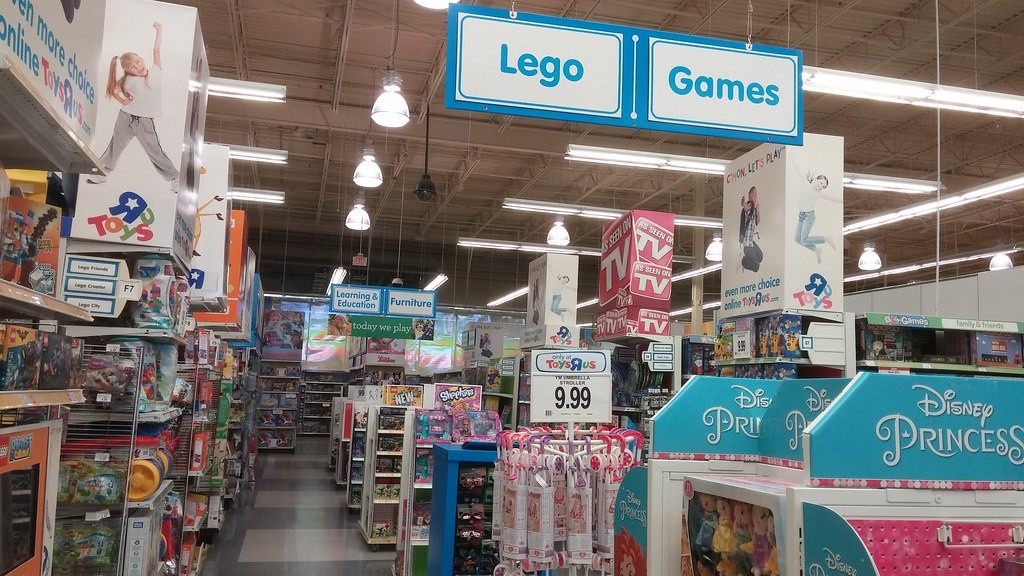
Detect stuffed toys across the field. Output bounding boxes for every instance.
[694,492,779,576]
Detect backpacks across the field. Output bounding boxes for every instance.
[739,201,760,243]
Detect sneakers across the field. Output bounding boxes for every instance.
[86,175,107,184]
[170,180,179,192]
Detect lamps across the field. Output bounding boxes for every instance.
[370,0,411,128]
[353,67,383,188]
[422,205,449,291]
[705,228,722,262]
[858,239,882,270]
[502,197,723,229]
[546,215,571,246]
[233,186,286,204]
[843,241,1024,283]
[345,189,371,231]
[563,144,946,193]
[670,262,725,317]
[208,77,288,103]
[988,239,1014,271]
[843,171,1024,238]
[325,201,347,295]
[204,141,288,167]
[802,65,1024,118]
[457,237,697,265]
[486,284,599,326]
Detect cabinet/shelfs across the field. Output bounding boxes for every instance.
[297,380,347,435]
[482,356,514,430]
[596,333,715,461]
[512,347,592,433]
[428,443,525,576]
[714,312,1024,380]
[345,386,371,516]
[334,385,359,491]
[346,364,406,384]
[391,382,503,576]
[0,255,257,576]
[463,360,488,391]
[356,384,422,552]
[613,379,1024,576]
[327,386,343,470]
[256,368,300,453]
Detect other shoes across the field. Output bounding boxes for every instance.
[816,247,822,264]
[740,244,745,256]
[827,235,836,250]
[736,256,745,275]
